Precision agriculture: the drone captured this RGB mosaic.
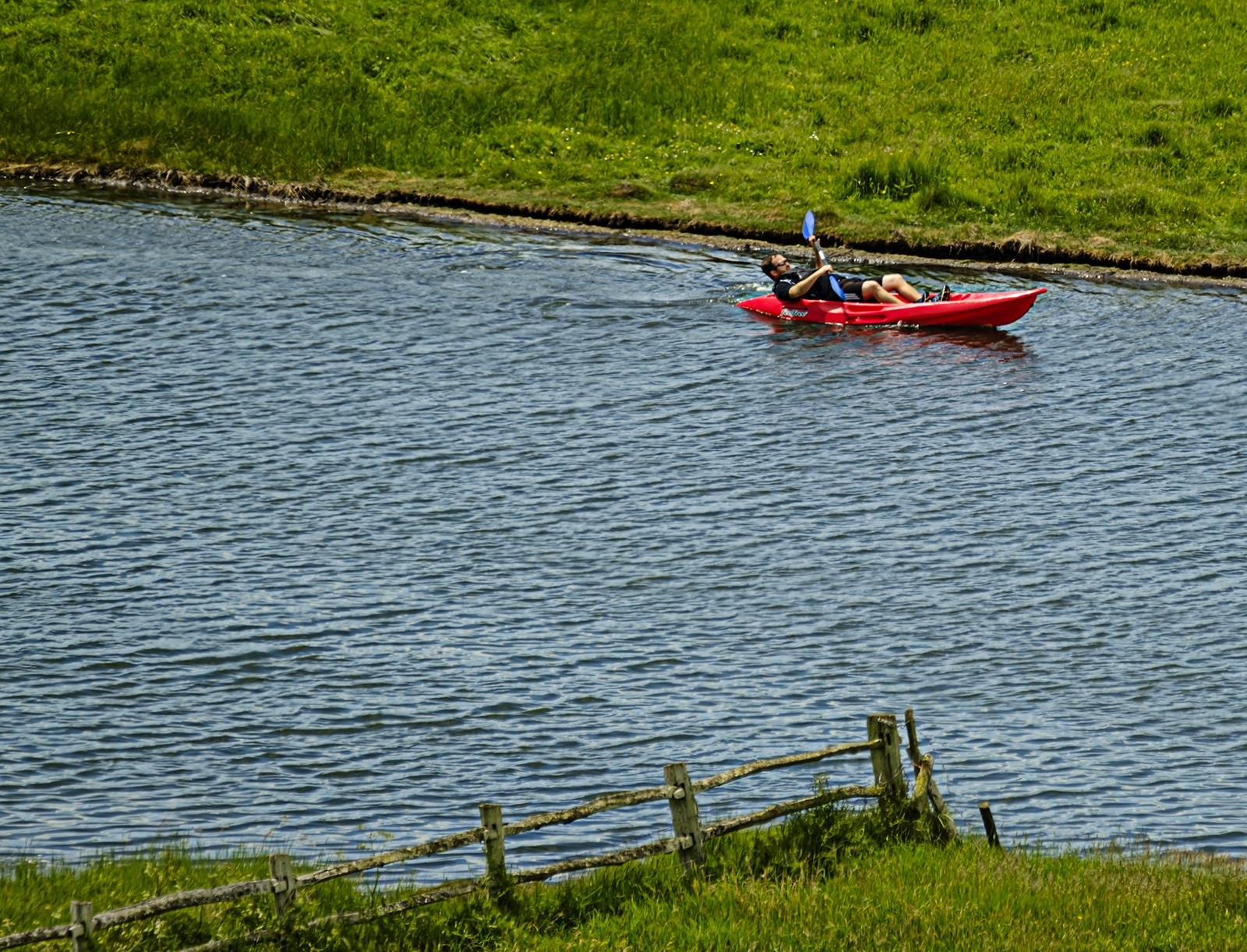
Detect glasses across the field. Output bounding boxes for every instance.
[776,259,792,268]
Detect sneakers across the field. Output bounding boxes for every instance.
[930,285,950,302]
[916,290,929,303]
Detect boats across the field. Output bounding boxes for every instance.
[733,285,1048,330]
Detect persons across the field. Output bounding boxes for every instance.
[761,252,951,305]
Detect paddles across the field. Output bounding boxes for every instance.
[802,211,846,300]
